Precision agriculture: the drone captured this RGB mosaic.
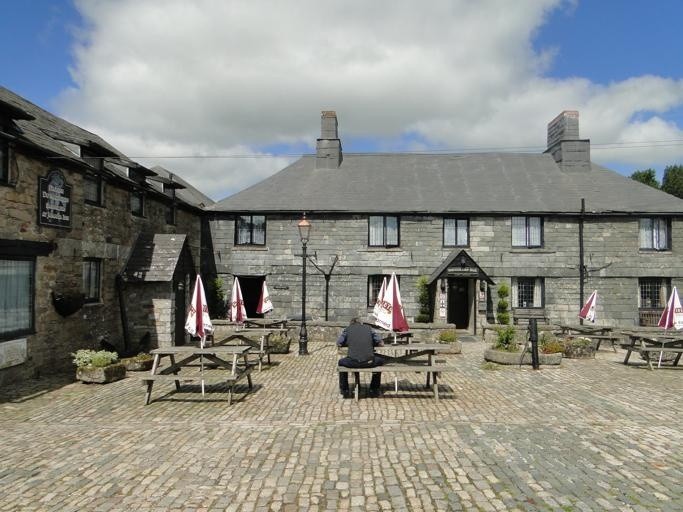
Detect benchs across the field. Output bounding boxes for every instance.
[334,328,462,404]
[554,323,682,373]
[137,317,293,408]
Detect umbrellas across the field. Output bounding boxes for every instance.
[655,286,682,369]
[375,272,408,344]
[257,278,272,330]
[228,276,247,346]
[373,277,388,318]
[577,289,597,328]
[183,274,212,397]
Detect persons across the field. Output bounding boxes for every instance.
[336,318,385,397]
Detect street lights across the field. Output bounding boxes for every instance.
[293,211,315,356]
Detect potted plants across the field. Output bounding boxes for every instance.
[68,349,155,384]
[484,285,561,365]
